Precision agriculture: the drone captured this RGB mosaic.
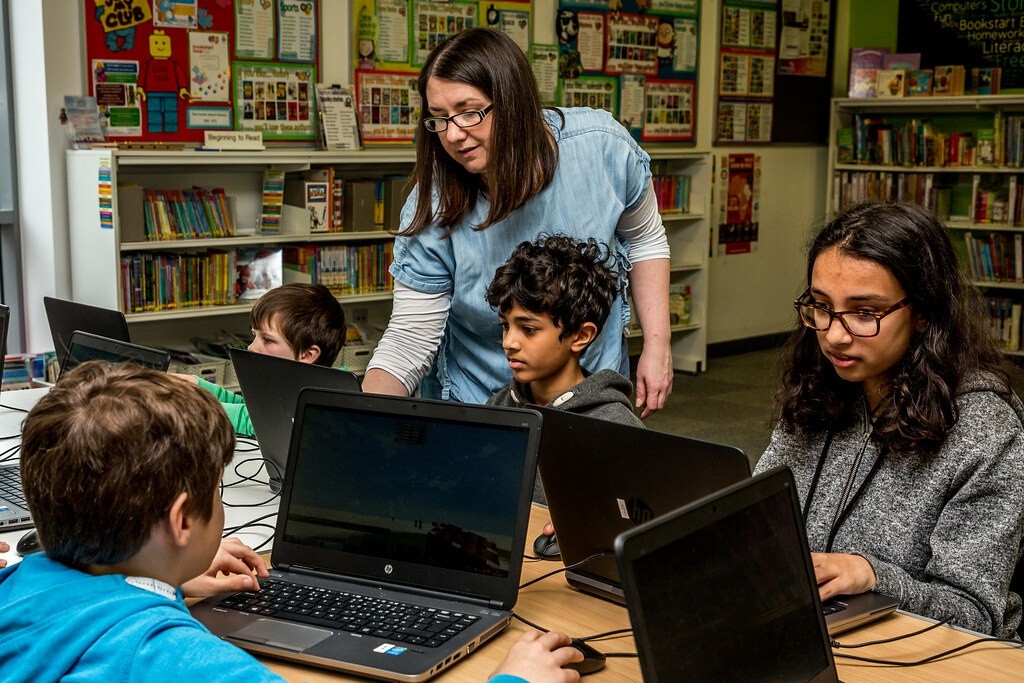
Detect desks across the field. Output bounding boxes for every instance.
[0,387,1024,683]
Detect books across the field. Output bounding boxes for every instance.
[651,174,690,213]
[834,172,951,222]
[964,231,1024,284]
[282,242,395,297]
[848,47,1001,97]
[121,248,237,315]
[837,114,995,167]
[982,294,1021,352]
[117,184,238,243]
[1001,113,1024,170]
[971,173,1024,226]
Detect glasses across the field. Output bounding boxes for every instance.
[794,286,909,338]
[424,103,492,132]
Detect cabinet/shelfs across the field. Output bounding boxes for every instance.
[63,146,707,377]
[824,95,1024,358]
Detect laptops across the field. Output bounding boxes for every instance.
[0,296,172,533]
[186,348,900,683]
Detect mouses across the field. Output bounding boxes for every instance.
[17,529,42,556]
[532,532,562,561]
[552,637,606,674]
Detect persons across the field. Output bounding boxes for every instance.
[751,200,1024,646]
[0,358,584,683]
[164,280,347,437]
[484,226,649,506]
[361,26,673,419]
